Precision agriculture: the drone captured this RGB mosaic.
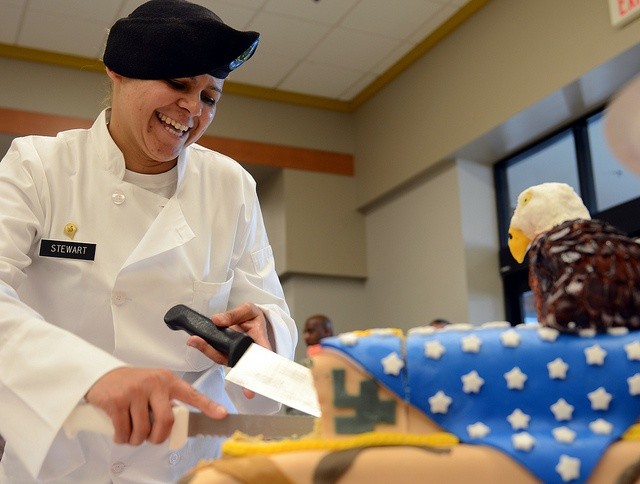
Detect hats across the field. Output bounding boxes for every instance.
[105,0,260,80]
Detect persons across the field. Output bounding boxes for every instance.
[305,314,333,346]
[2,0,298,484]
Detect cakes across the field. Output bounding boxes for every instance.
[186,182,640,483]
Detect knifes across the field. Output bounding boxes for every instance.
[162,303,321,417]
[63,404,315,451]
